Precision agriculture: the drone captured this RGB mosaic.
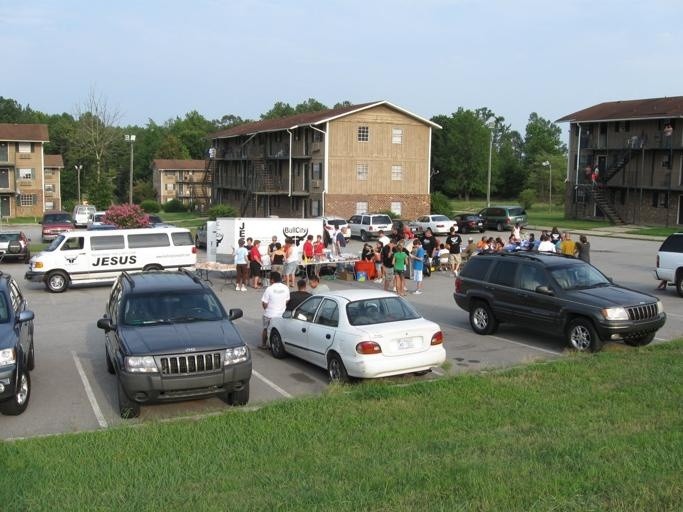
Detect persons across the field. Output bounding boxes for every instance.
[323,224,351,274]
[235,233,324,291]
[362,224,463,296]
[463,223,590,264]
[282,280,313,318]
[584,164,600,192]
[632,135,640,148]
[664,124,673,148]
[258,272,290,348]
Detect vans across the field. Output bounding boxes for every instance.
[650,231,682,300]
[477,205,528,232]
[22,225,198,295]
[71,205,97,228]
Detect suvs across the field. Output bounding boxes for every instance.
[93,263,252,424]
[0,269,40,421]
[319,214,352,244]
[447,246,669,356]
[37,209,75,243]
[343,212,394,242]
[0,228,32,266]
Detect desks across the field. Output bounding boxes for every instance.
[293,252,362,285]
[190,262,237,291]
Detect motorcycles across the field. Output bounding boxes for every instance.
[476,214,488,233]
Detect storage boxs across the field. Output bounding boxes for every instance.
[341,271,353,282]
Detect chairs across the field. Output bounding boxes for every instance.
[438,252,451,271]
[460,253,469,271]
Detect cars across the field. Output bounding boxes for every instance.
[194,222,207,251]
[389,217,424,235]
[260,287,448,388]
[449,210,489,234]
[412,213,459,236]
[83,212,175,232]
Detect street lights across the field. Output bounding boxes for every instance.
[73,163,83,203]
[123,133,136,205]
[628,134,641,226]
[663,123,674,228]
[485,121,495,207]
[519,151,525,165]
[540,159,554,213]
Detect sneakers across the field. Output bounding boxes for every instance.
[392,285,424,295]
[239,287,247,292]
[373,276,385,284]
[289,284,295,288]
[234,286,240,291]
[250,285,261,289]
[257,343,267,350]
[438,267,459,278]
